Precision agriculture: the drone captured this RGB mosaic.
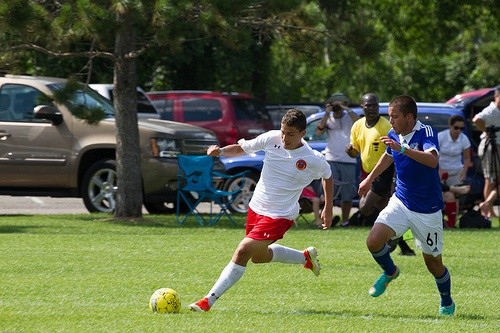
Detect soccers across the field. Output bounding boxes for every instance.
[150,288,181,314]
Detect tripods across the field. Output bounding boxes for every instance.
[464,133,500,224]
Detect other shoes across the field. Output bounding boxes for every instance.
[400,251,416,255]
[331,215,340,227]
[316,224,323,229]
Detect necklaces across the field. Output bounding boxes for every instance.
[365,120,376,128]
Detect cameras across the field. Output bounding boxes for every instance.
[330,104,342,113]
[486,125,500,133]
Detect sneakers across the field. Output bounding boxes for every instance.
[189,297,210,312]
[303,246,320,277]
[439,299,455,315]
[369,266,400,297]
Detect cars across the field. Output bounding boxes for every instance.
[0,74,217,216]
[214,86,499,219]
[443,87,496,104]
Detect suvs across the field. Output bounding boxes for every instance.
[1,82,161,118]
[265,106,324,131]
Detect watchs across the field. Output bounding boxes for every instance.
[404,144,410,154]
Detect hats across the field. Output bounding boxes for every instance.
[326,93,352,105]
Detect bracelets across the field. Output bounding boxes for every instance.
[318,126,324,131]
[220,148,224,154]
[400,145,405,153]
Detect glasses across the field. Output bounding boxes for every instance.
[452,125,465,131]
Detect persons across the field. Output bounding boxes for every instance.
[471,88,500,219]
[436,114,471,217]
[317,94,360,226]
[345,93,413,255]
[357,95,455,315]
[188,108,334,312]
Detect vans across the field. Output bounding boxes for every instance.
[147,90,275,148]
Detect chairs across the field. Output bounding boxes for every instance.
[295,179,349,228]
[174,155,249,228]
